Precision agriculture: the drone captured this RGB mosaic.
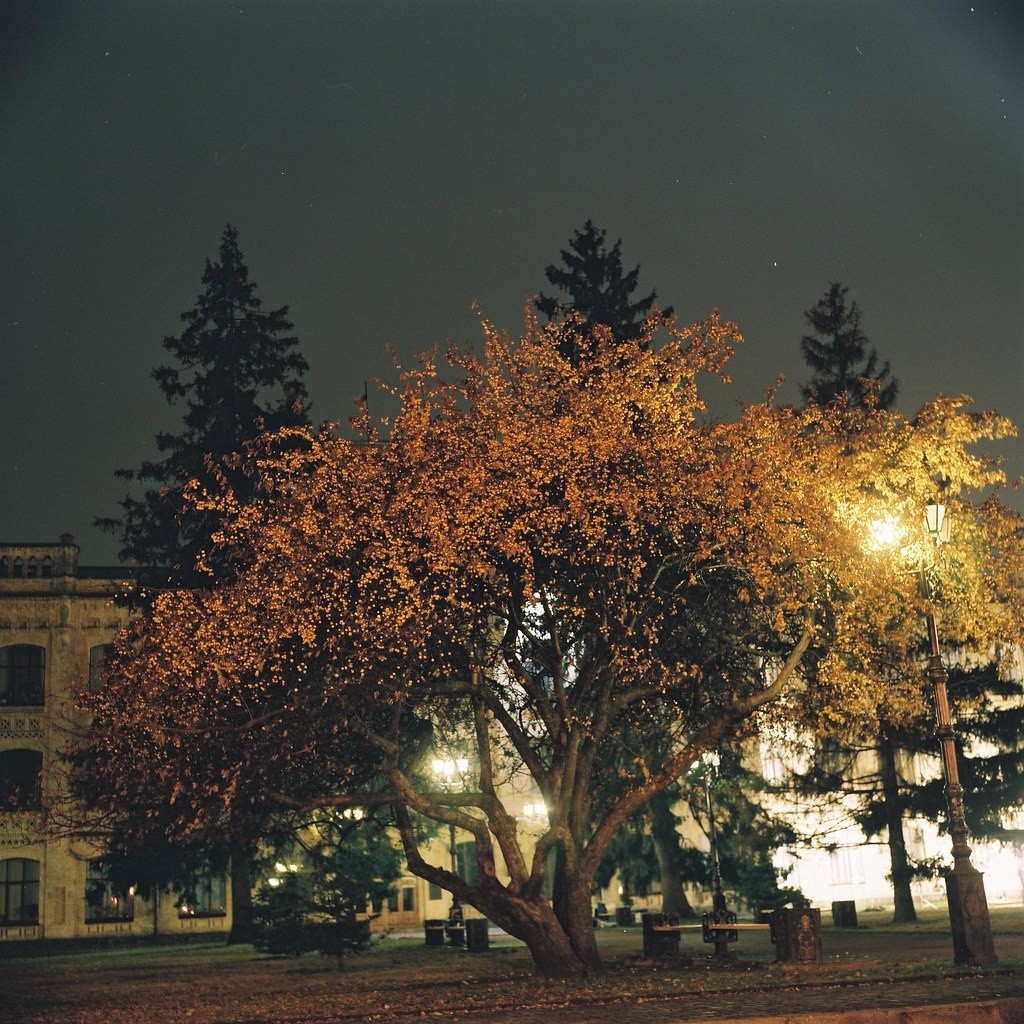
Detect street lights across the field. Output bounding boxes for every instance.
[688,751,733,911]
[869,499,999,969]
[432,752,468,946]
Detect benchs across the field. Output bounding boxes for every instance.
[642,907,823,963]
[754,900,857,927]
[424,919,488,950]
[592,906,646,927]
